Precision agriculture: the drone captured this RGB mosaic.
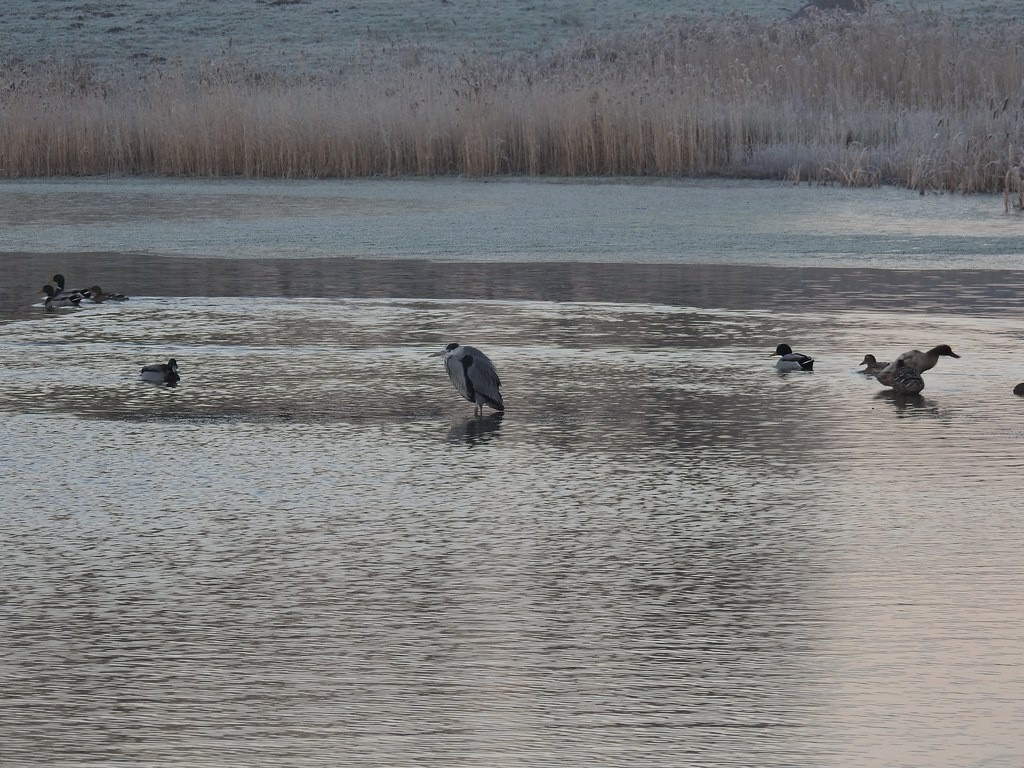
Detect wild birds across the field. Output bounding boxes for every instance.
[859,344,962,396]
[769,344,814,370]
[35,274,129,309]
[428,343,505,417]
[139,358,179,374]
